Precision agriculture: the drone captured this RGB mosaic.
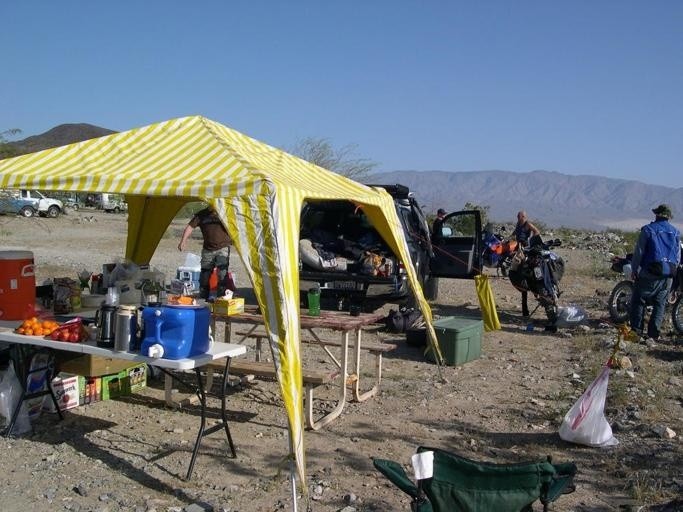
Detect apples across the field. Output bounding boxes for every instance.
[50,324,88,342]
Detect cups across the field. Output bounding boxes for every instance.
[105,287,119,307]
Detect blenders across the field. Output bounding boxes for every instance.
[138,271,164,306]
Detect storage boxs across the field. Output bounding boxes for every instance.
[176,266,201,290]
[42,372,80,414]
[426,315,484,366]
[101,372,126,401]
[126,362,148,395]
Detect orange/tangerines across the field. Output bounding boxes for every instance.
[15,316,59,336]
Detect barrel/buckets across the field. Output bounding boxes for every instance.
[141,307,210,359]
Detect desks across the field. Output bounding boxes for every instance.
[0,318,247,481]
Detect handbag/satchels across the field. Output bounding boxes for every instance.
[387,307,421,333]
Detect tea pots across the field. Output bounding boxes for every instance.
[94,304,116,347]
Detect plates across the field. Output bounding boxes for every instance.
[167,304,200,307]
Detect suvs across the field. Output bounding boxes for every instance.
[18,188,61,217]
[299,183,483,315]
[86,192,127,214]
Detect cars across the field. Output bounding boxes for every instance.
[64,192,83,212]
[0,190,33,219]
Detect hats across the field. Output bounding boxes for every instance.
[437,209,447,214]
[652,204,672,219]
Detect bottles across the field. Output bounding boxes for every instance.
[308,288,321,315]
[113,304,137,354]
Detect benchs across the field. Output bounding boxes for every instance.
[235,328,398,384]
[198,359,332,421]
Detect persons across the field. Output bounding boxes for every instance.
[509,211,539,251]
[630,205,681,339]
[433,208,447,235]
[178,204,233,298]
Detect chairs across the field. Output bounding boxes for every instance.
[373,446,577,512]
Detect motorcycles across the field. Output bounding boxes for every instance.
[608,249,682,334]
[521,238,566,333]
